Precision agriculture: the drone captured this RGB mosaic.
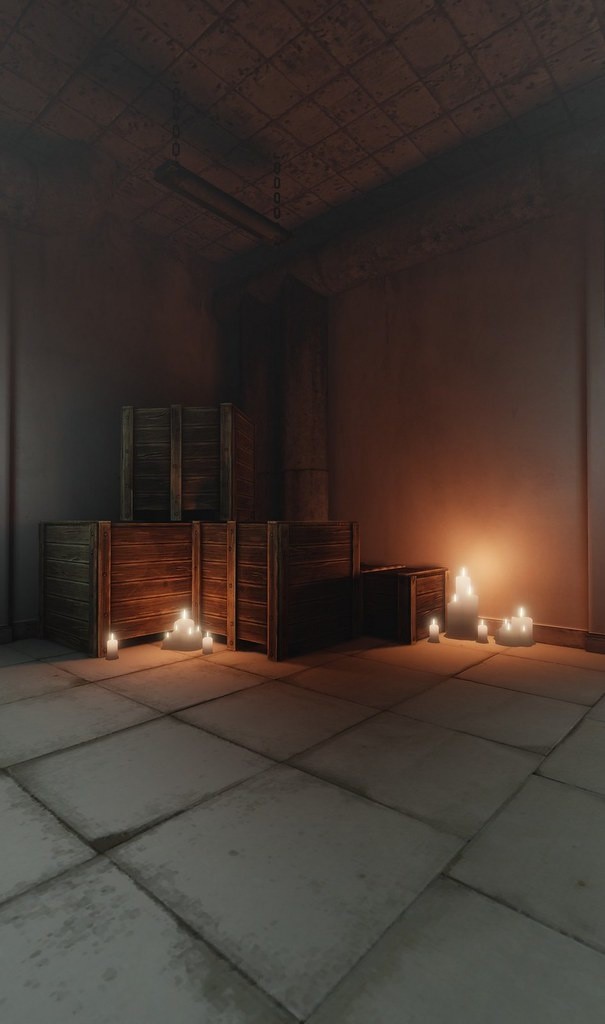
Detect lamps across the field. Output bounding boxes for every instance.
[138,83,296,253]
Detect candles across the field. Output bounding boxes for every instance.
[105,632,119,661]
[443,565,480,642]
[427,617,441,644]
[494,605,535,648]
[200,630,215,656]
[476,618,489,644]
[161,608,203,652]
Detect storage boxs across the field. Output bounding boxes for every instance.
[196,521,363,662]
[35,519,197,656]
[363,565,449,645]
[119,402,256,521]
